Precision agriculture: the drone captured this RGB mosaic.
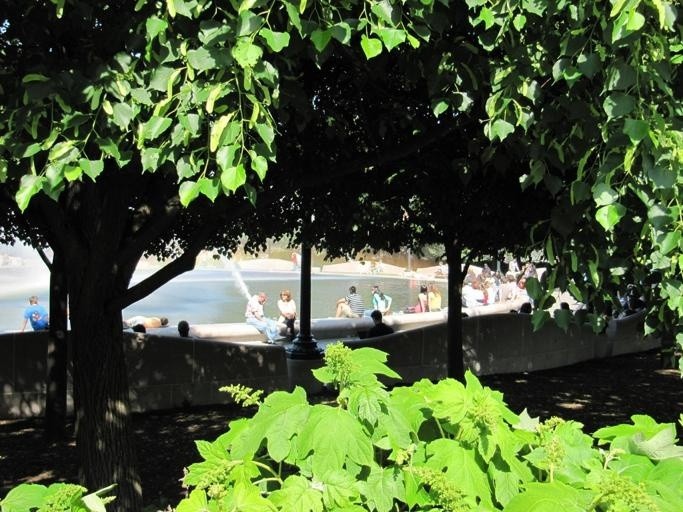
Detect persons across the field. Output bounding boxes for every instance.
[123,316,170,329]
[365,310,394,338]
[405,285,428,313]
[335,287,366,318]
[178,320,202,340]
[434,258,521,308]
[245,293,280,345]
[365,286,393,317]
[427,283,442,312]
[276,291,300,338]
[18,296,50,338]
